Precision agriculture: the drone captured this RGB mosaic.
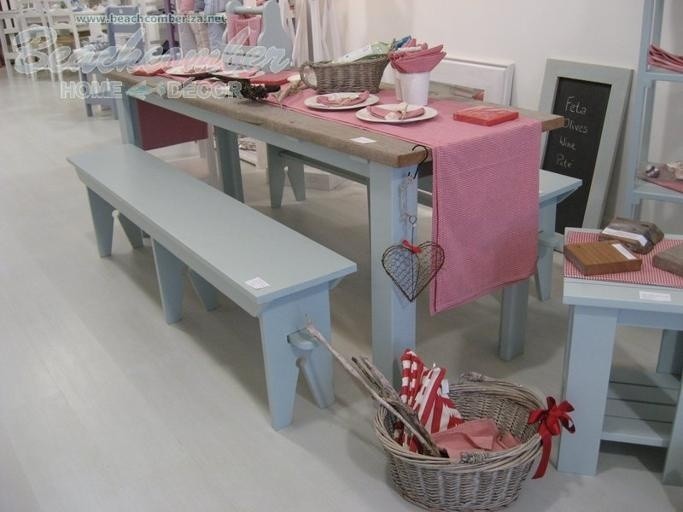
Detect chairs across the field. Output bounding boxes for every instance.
[0,0,143,119]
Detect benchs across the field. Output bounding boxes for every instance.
[262,140,583,302]
[66,138,359,428]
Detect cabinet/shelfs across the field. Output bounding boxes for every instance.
[615,0,681,218]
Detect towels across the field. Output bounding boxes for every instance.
[388,38,446,73]
[316,88,369,106]
[366,104,425,121]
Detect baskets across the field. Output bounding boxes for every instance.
[299,52,392,98]
[371,371,556,512]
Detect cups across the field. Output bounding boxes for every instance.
[392,71,402,101]
[398,72,430,106]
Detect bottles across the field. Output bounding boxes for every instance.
[331,35,412,66]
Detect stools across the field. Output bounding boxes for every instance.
[554,224,682,487]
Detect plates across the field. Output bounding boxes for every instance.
[356,103,440,125]
[288,74,306,86]
[165,64,221,79]
[304,91,380,111]
[211,67,264,81]
